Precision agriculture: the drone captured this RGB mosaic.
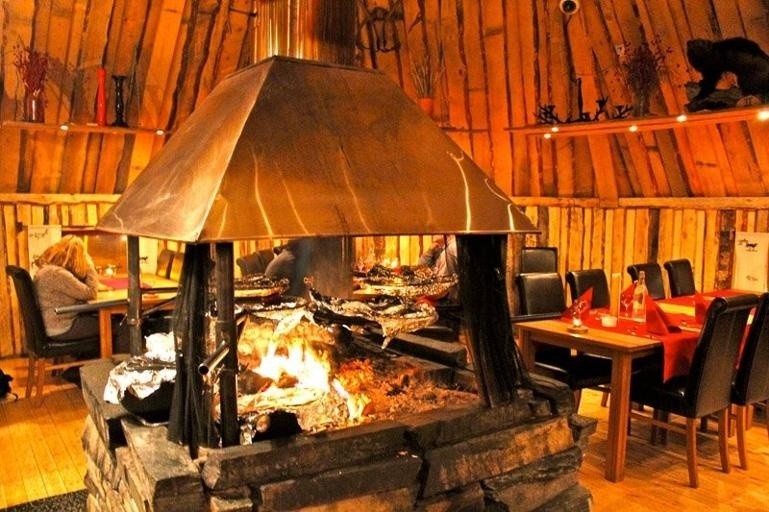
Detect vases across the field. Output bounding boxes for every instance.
[639,90,653,126]
[95,67,107,125]
[23,85,46,124]
[110,73,128,127]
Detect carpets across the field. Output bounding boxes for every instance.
[2,488,91,510]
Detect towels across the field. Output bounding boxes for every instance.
[695,290,715,329]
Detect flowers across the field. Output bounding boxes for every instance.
[9,36,51,121]
[598,34,684,89]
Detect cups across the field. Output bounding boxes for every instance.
[602,316,618,328]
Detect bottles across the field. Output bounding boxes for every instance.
[633,272,649,320]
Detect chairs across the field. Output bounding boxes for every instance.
[5,249,186,408]
[699,294,766,471]
[512,243,698,416]
[629,294,757,489]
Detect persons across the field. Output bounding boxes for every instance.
[261,237,300,288]
[30,234,99,388]
[416,234,457,277]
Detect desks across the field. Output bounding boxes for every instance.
[231,260,434,307]
